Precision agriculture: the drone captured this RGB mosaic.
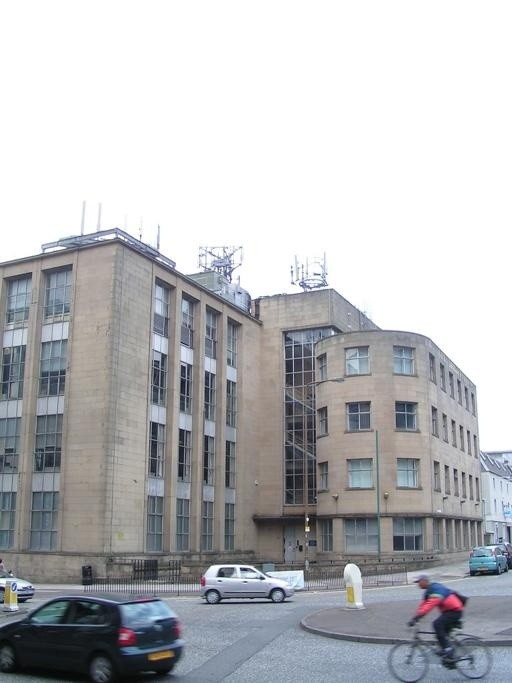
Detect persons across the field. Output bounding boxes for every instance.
[1,558,5,569]
[404,574,468,669]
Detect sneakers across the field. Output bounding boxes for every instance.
[434,646,455,656]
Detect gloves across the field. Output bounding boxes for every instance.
[406,618,416,627]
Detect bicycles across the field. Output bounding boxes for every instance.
[386,617,494,682]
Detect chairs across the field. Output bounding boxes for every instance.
[43,604,79,630]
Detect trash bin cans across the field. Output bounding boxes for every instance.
[82,565,92,585]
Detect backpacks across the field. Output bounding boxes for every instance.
[454,591,469,604]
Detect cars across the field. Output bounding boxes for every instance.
[0,593,183,683]
[469,542,511,576]
[0,569,34,603]
[200,564,293,604]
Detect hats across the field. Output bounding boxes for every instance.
[413,574,429,583]
